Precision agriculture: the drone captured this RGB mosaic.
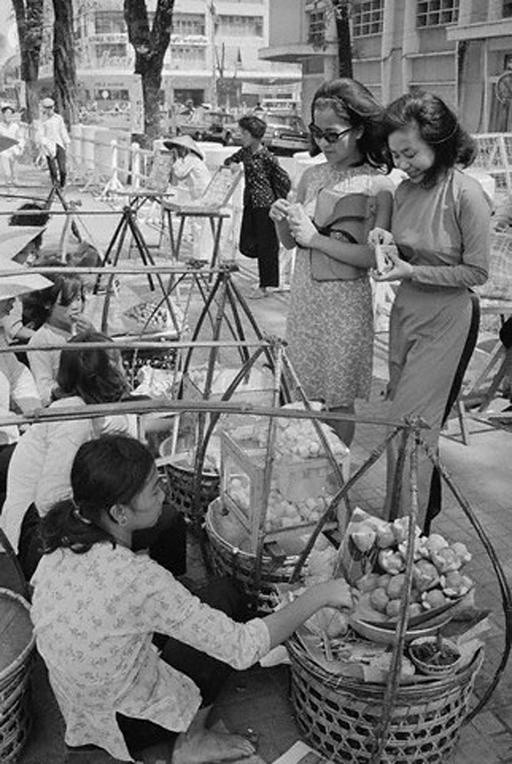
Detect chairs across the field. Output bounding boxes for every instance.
[157,164,244,261]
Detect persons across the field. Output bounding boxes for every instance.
[290,104,297,115]
[267,76,395,449]
[170,95,194,115]
[254,103,263,111]
[367,92,493,536]
[1,97,95,489]
[1,336,200,598]
[162,136,213,269]
[224,117,292,295]
[29,433,355,764]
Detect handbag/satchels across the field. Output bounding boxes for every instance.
[311,187,367,282]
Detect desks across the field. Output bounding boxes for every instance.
[107,188,175,259]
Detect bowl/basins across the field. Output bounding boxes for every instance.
[409,634,461,676]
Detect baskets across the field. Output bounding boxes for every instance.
[159,437,308,615]
[0,587,35,764]
[284,636,485,764]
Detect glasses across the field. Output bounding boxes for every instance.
[309,123,353,142]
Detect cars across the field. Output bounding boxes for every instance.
[148,102,316,154]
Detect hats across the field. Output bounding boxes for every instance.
[0,226,54,300]
[163,135,204,160]
